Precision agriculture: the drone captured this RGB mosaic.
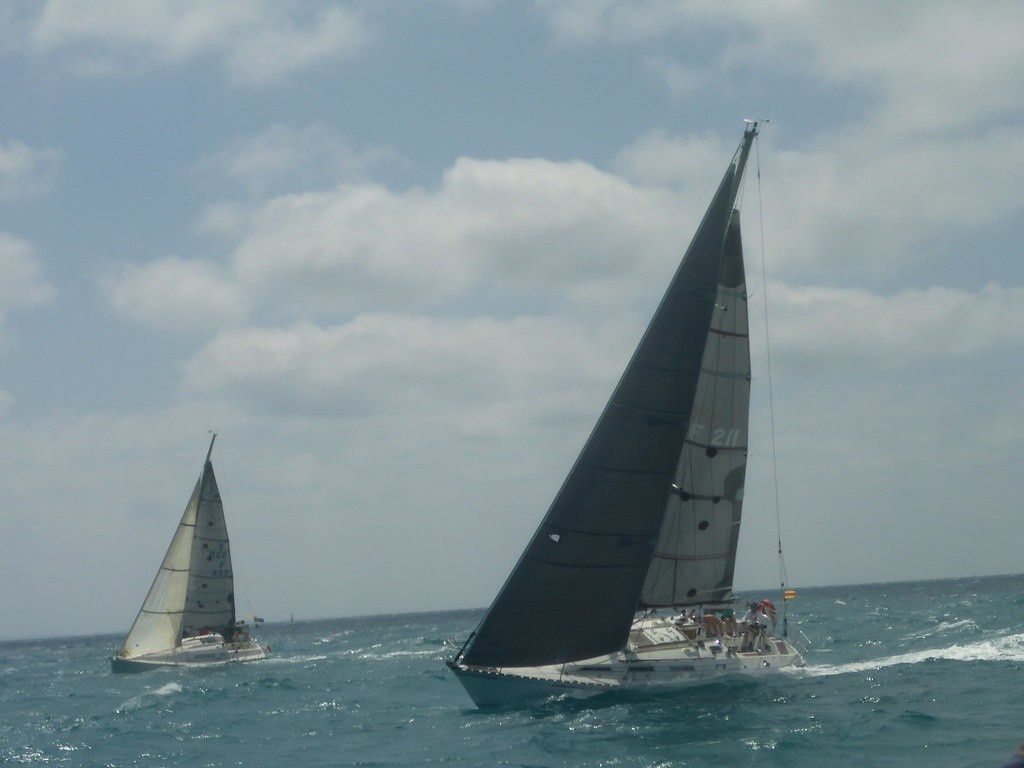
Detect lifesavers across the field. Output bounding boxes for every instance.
[758,600,776,625]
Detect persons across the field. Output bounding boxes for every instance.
[684,601,770,656]
[181,620,247,645]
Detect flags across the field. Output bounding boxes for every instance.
[783,590,799,600]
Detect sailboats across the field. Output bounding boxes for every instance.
[252,617,265,628]
[443,116,810,716]
[106,433,271,676]
